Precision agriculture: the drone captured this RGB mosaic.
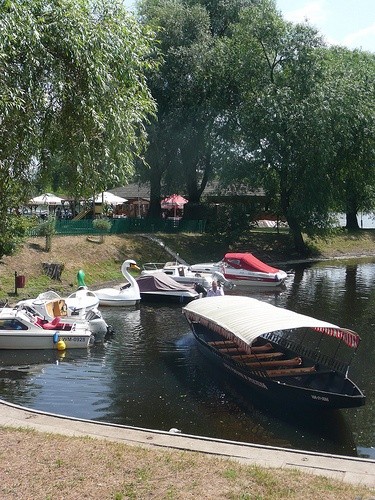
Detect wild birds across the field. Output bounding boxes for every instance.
[169,427,182,434]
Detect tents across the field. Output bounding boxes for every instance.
[27,192,69,215]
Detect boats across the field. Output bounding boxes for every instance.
[191,252,288,287]
[183,294,368,410]
[66,269,100,313]
[120,261,229,302]
[0,290,114,350]
[94,258,143,306]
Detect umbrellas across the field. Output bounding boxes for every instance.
[79,191,128,219]
[161,193,188,217]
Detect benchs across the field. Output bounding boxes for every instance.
[208,339,317,376]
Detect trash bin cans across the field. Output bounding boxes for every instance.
[16,276,26,288]
